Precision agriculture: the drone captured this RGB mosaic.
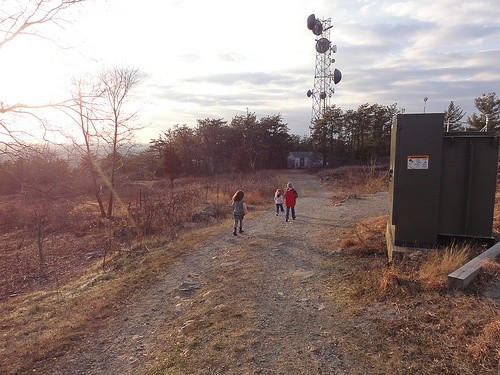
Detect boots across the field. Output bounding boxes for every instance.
[276,213,279,216]
[232,227,237,235]
[239,226,244,233]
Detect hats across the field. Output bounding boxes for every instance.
[287,182,292,188]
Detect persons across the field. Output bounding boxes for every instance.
[284,181,299,222]
[273,189,284,216]
[231,190,249,236]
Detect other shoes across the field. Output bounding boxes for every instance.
[293,217,296,220]
[285,219,288,222]
